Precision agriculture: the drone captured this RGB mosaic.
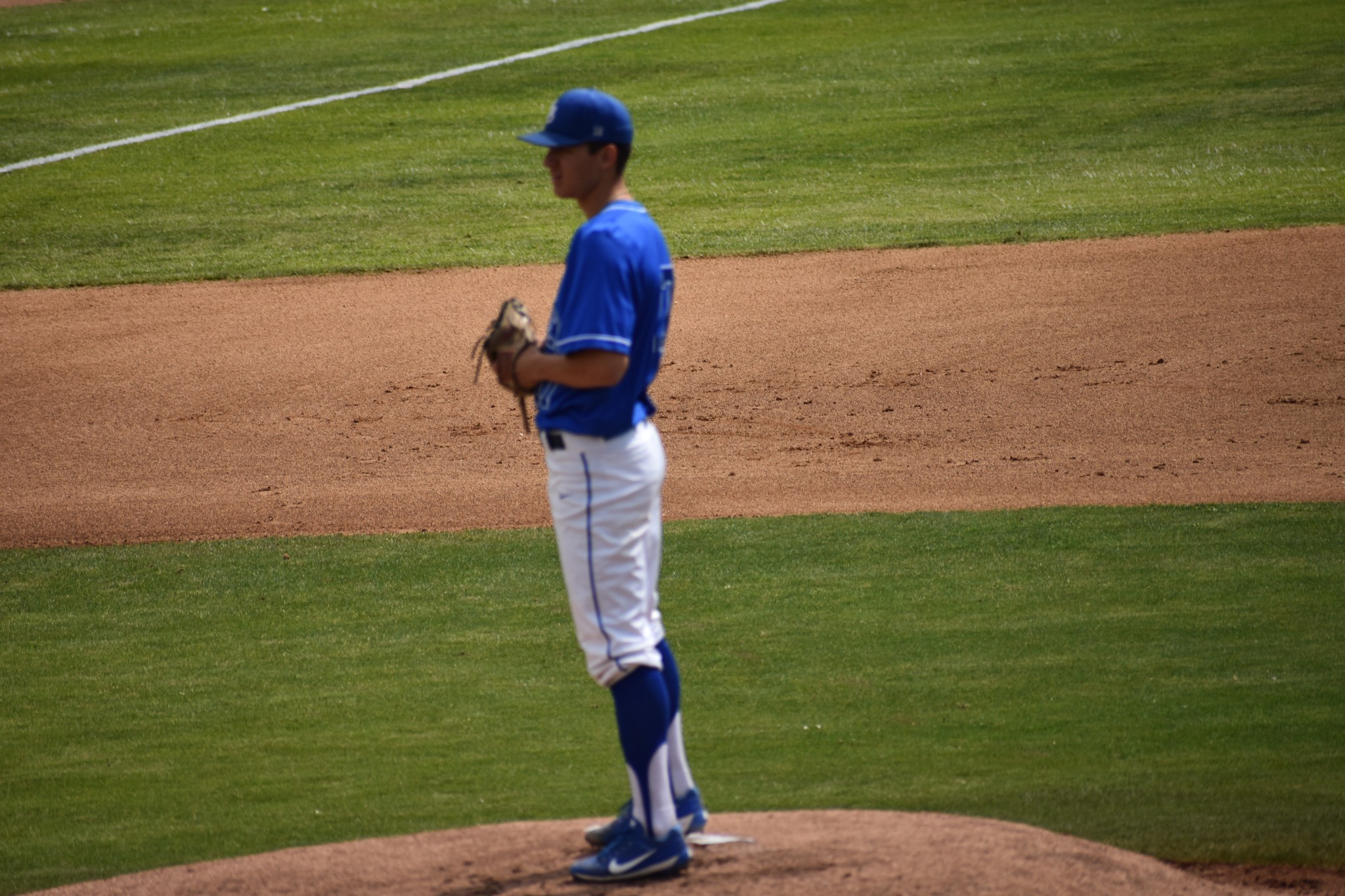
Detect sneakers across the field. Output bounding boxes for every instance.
[585,785,709,845]
[568,818,690,882]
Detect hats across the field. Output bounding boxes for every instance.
[517,89,637,148]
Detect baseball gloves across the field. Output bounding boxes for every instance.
[483,297,538,398]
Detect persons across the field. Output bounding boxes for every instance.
[471,90,710,886]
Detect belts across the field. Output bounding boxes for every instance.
[546,435,565,450]
